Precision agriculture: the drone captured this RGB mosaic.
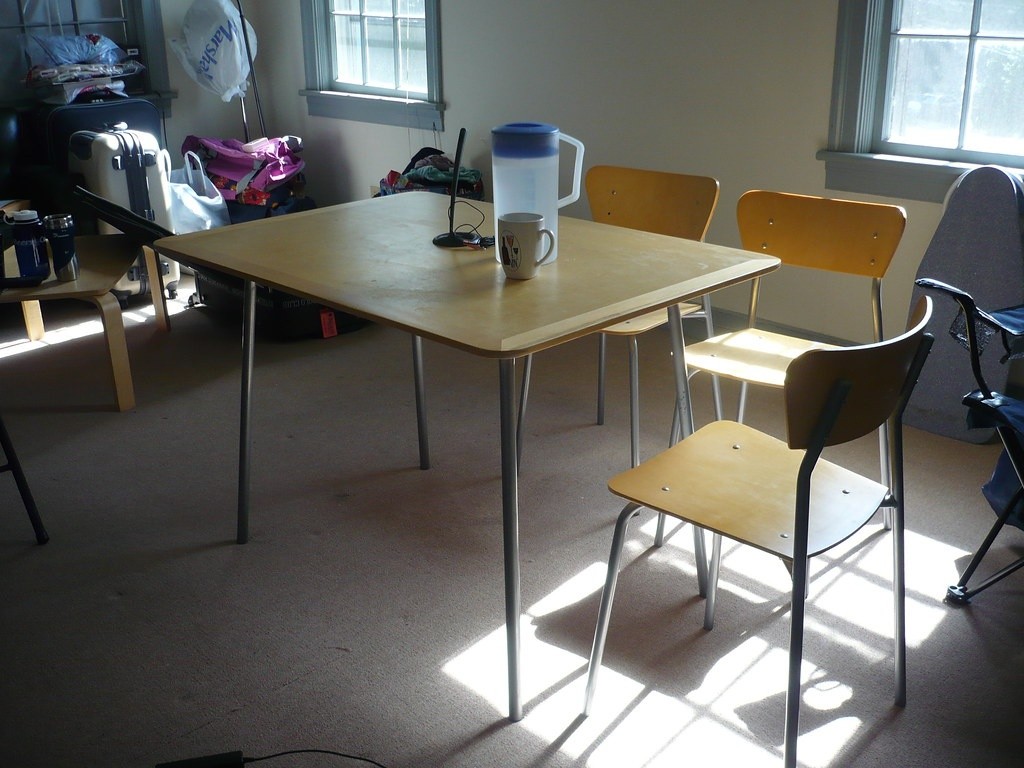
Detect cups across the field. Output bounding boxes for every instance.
[497,213,555,279]
[3,210,52,281]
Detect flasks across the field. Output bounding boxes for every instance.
[44,213,79,281]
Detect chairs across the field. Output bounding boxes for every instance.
[512,165,936,768]
[916,279,1024,610]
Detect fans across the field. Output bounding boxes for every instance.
[170,0,257,145]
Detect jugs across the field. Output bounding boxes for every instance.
[490,122,585,265]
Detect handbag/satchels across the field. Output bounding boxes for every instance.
[181,130,307,206]
[161,148,231,275]
[167,0,258,102]
[16,30,128,71]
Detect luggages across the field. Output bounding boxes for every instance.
[188,270,374,343]
[66,121,181,309]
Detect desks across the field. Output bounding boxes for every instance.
[0,233,172,413]
[152,191,781,722]
